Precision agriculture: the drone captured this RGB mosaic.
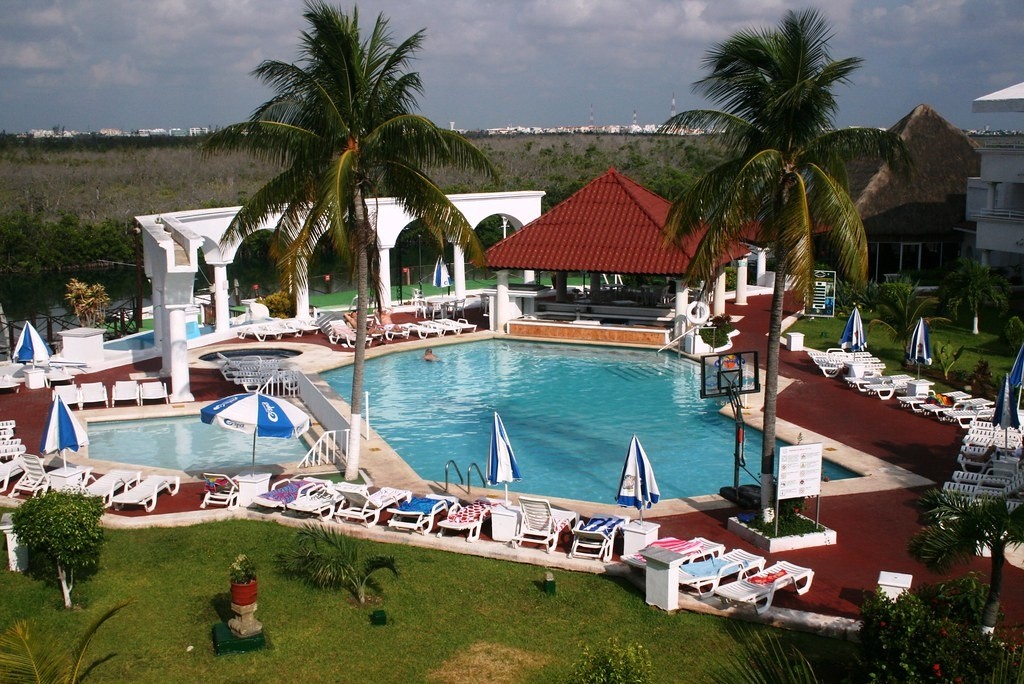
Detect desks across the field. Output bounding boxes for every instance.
[603,283,626,300]
[424,295,460,319]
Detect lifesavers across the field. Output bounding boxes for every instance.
[687,301,710,325]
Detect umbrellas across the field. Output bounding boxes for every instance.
[992,342,1024,459]
[432,255,451,297]
[838,306,867,361]
[200,392,311,479]
[12,321,53,370]
[485,412,522,507]
[615,433,660,526]
[908,317,932,379]
[39,393,88,472]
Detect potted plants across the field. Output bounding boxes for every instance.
[229,552,259,605]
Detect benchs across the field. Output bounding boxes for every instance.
[884,273,901,283]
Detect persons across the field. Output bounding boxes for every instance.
[344,311,385,335]
[376,306,403,332]
[424,348,435,359]
[967,435,1024,463]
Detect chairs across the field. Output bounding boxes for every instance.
[81,469,142,509]
[436,497,512,543]
[327,289,478,349]
[807,347,873,359]
[919,397,996,421]
[112,381,140,409]
[333,486,413,529]
[0,374,20,395]
[968,428,1023,441]
[677,548,767,601]
[111,475,180,513]
[961,444,1022,461]
[237,317,320,341]
[51,384,81,411]
[386,494,460,536]
[952,468,1024,486]
[80,382,109,409]
[938,493,1024,518]
[815,362,887,378]
[957,453,1024,473]
[216,352,301,393]
[942,404,998,429]
[566,513,630,562]
[201,472,240,511]
[286,481,368,521]
[844,370,915,392]
[252,476,332,512]
[714,560,815,617]
[619,534,727,571]
[897,390,973,412]
[140,381,169,406]
[863,379,935,401]
[944,478,1024,498]
[511,496,580,554]
[0,419,52,500]
[962,435,1024,448]
[969,419,1023,433]
[813,355,882,366]
[46,366,75,390]
